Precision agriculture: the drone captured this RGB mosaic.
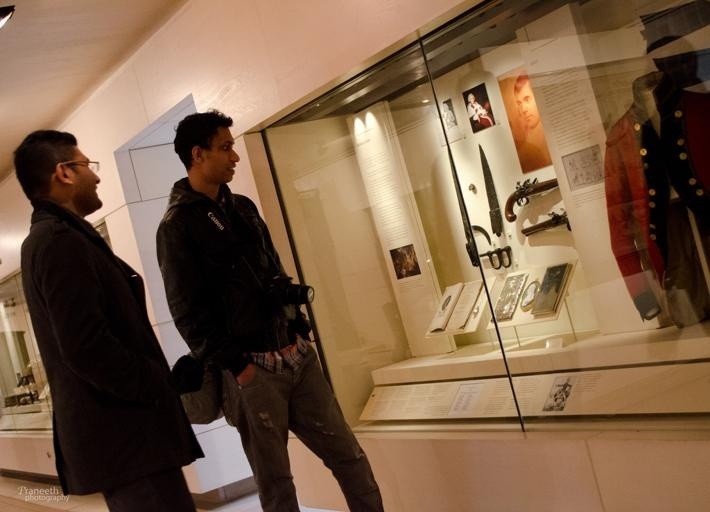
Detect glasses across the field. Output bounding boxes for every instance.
[63,160,100,174]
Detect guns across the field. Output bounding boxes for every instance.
[520,209,571,234]
[505,177,559,222]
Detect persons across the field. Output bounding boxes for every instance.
[468,93,494,127]
[12,128,207,512]
[603,70,709,329]
[443,102,456,127]
[513,69,553,173]
[155,108,385,512]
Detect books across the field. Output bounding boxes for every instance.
[490,272,529,322]
[429,280,484,332]
[530,262,572,317]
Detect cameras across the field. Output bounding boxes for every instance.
[265,275,315,311]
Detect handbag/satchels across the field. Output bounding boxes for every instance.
[172,351,228,426]
